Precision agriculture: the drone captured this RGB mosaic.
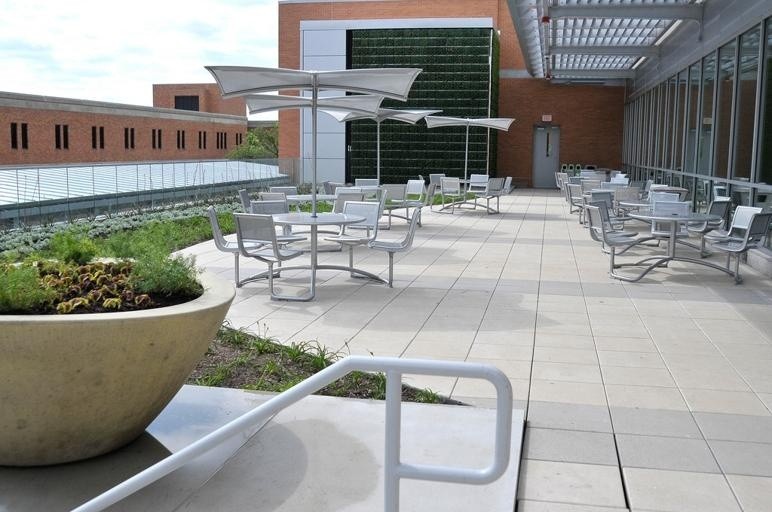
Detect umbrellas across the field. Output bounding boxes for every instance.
[424,115,516,202]
[316,108,444,202]
[203,65,424,264]
[241,93,386,116]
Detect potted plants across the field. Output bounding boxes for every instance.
[1,252,236,469]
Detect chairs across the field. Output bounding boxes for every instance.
[554,164,772,283]
[206,178,426,303]
[418,173,512,214]
